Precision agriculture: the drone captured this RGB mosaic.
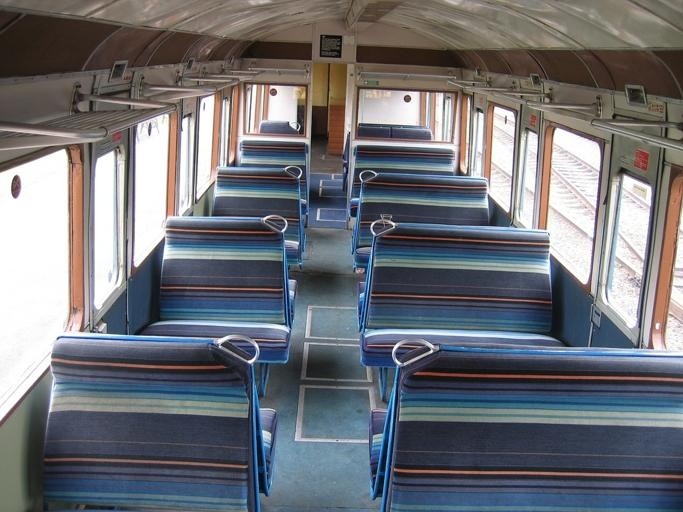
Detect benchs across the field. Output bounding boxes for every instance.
[359,127,432,140]
[360,220,565,400]
[352,170,493,269]
[347,145,456,229]
[138,217,299,397]
[359,281,365,314]
[211,166,302,271]
[40,332,278,511]
[359,123,425,128]
[259,120,301,134]
[343,132,350,191]
[368,339,683,510]
[238,140,309,227]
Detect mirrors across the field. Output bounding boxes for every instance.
[356,86,458,144]
[244,83,307,137]
